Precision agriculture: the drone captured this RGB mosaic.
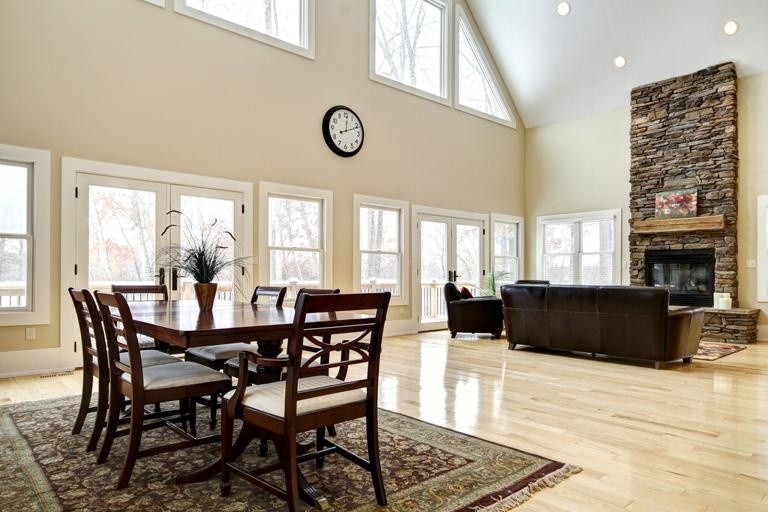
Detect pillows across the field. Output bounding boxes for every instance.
[460,286,473,299]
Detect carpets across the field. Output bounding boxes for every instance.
[1,390,582,512]
[694,340,748,362]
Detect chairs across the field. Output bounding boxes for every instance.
[442,282,503,340]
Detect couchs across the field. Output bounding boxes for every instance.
[499,283,705,368]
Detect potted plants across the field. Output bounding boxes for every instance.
[157,203,251,313]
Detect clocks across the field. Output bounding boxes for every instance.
[322,106,365,157]
